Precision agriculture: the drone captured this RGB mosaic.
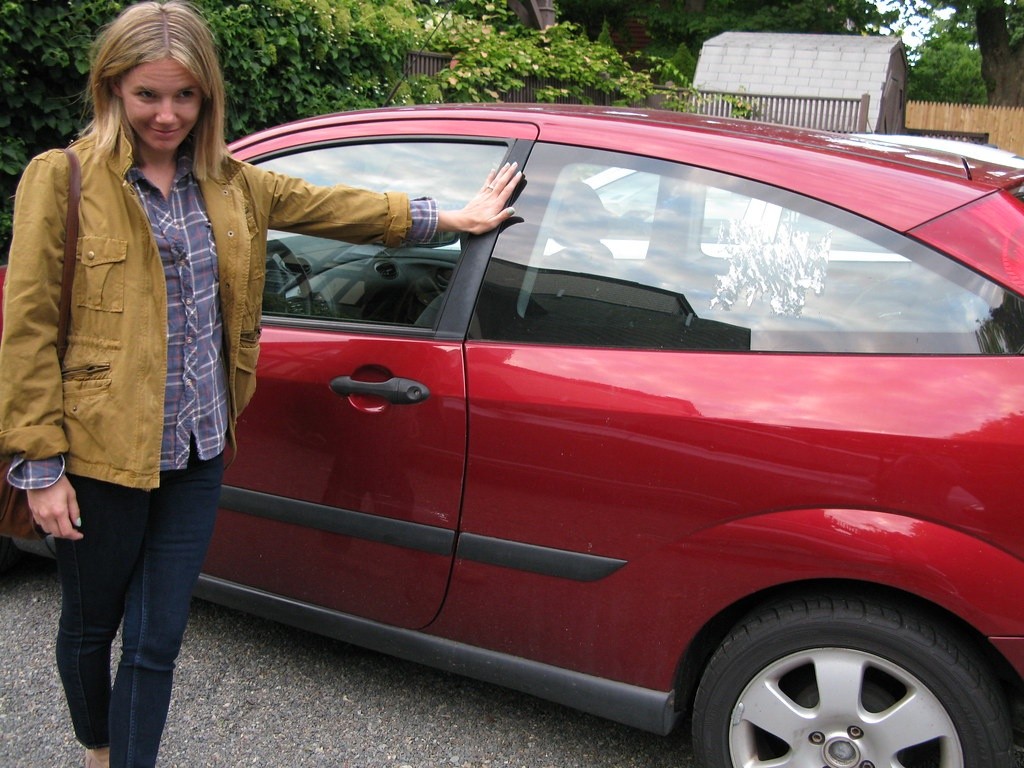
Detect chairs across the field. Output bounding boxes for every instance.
[411,207,535,335]
[532,180,614,342]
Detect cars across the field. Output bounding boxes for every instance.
[439,133,1024,293]
[0,100,1024,768]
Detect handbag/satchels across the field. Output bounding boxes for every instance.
[0,452,52,540]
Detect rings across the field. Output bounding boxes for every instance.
[487,185,493,190]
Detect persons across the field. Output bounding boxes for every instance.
[0,0,522,768]
